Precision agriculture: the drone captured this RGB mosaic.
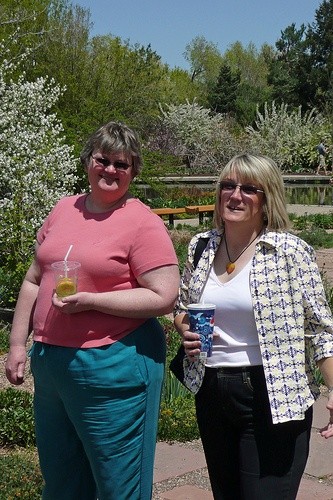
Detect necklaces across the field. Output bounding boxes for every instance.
[223,224,263,275]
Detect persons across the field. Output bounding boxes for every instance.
[6,122,180,500]
[316,139,328,176]
[169,152,333,500]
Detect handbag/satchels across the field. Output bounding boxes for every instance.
[168,343,186,387]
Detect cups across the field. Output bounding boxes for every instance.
[51,261,81,302]
[186,303,216,359]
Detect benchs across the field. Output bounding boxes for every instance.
[151,205,215,227]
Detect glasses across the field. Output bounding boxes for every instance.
[90,155,127,170]
[218,181,263,195]
